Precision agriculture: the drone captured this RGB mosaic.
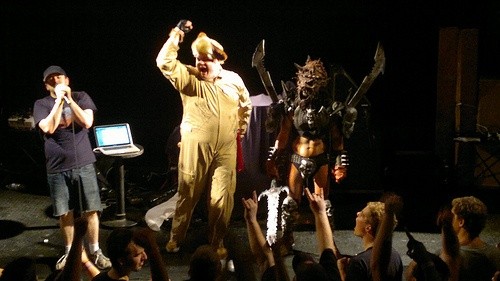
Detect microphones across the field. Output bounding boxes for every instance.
[63,95,71,105]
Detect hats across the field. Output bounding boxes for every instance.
[42,65,67,82]
[191,32,228,63]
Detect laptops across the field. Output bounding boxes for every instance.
[94,123,140,155]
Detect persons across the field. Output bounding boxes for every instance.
[265,59,350,260]
[33,66,113,270]
[156,19,251,253]
[0,187,500,281]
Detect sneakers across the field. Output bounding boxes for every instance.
[88,249,112,270]
[55,253,71,270]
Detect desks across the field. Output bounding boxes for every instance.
[8,111,44,144]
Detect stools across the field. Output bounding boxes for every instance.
[93,145,146,228]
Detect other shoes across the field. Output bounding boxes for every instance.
[283,237,296,248]
[166,240,181,256]
[220,257,235,272]
